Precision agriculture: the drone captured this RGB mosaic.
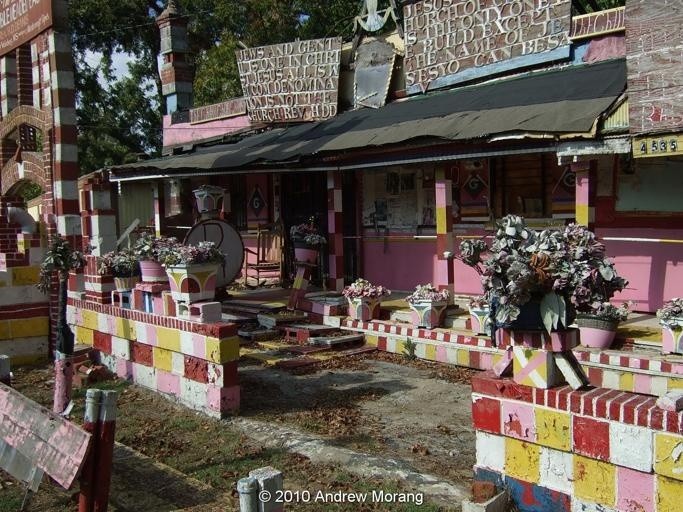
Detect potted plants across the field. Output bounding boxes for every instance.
[96,231,179,293]
[442,212,629,337]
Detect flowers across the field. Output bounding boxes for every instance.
[340,277,492,311]
[591,298,683,322]
[157,240,226,270]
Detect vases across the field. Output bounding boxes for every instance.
[161,262,218,305]
[345,295,489,336]
[576,313,683,355]
